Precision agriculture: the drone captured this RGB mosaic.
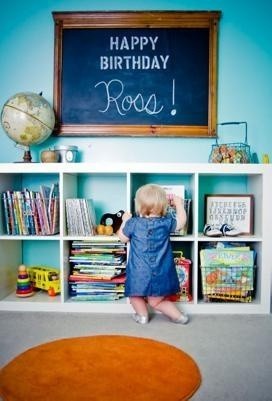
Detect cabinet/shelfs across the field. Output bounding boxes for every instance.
[1,162,270,315]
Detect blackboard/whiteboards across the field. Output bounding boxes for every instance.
[51,10,222,138]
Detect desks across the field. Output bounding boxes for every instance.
[1,335,202,401]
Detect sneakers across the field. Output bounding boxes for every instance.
[133,313,149,324]
[203,224,241,236]
[173,314,189,324]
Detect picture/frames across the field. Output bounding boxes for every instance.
[200,194,256,237]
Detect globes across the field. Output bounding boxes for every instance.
[0,92,55,163]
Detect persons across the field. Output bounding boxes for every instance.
[115,184,190,325]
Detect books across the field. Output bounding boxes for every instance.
[159,185,186,212]
[197,240,258,297]
[68,240,127,302]
[65,196,95,236]
[2,184,61,235]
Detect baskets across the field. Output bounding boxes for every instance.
[208,142,252,164]
[200,265,258,304]
[0,197,59,236]
